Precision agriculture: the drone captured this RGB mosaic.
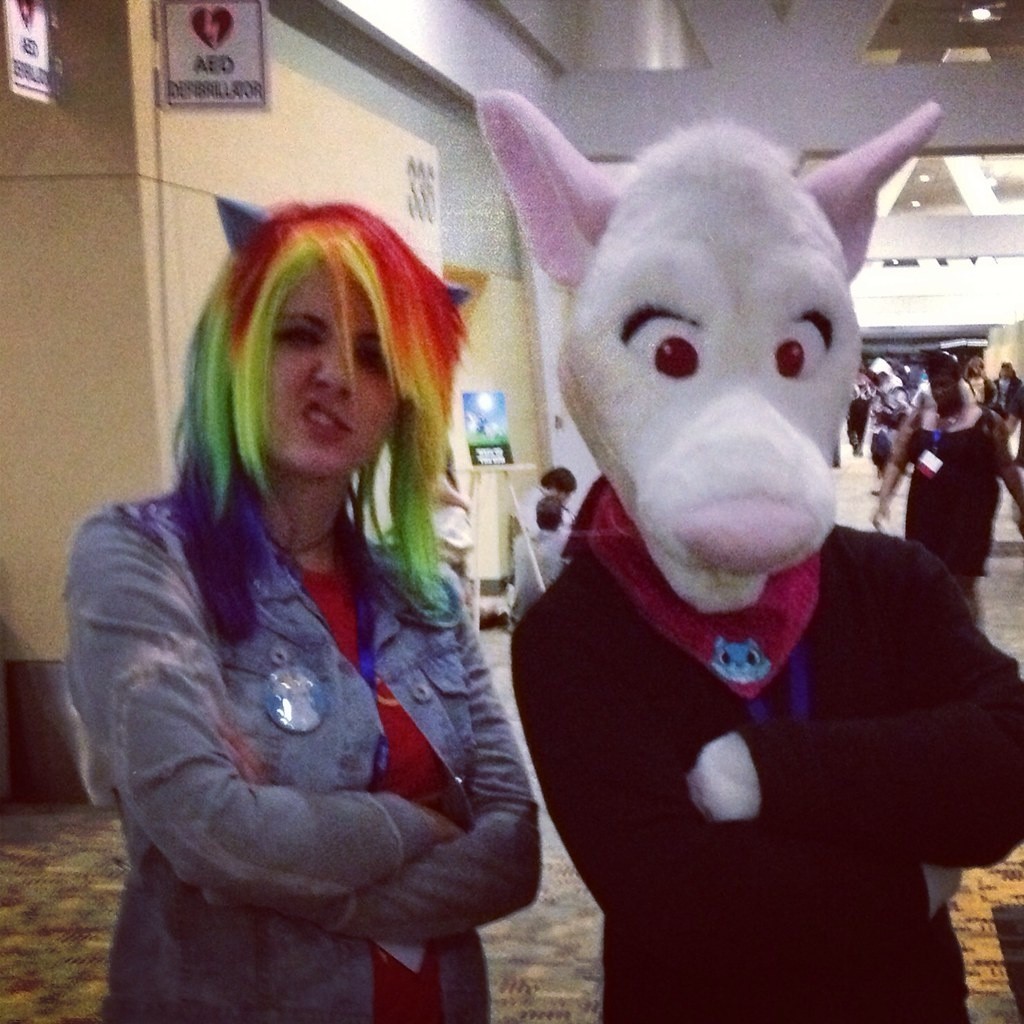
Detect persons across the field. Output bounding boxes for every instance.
[508,465,579,630]
[60,197,543,1023]
[845,347,1024,630]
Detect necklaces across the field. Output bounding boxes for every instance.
[290,553,379,688]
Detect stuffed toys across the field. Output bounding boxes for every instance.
[477,87,1024,1024]
